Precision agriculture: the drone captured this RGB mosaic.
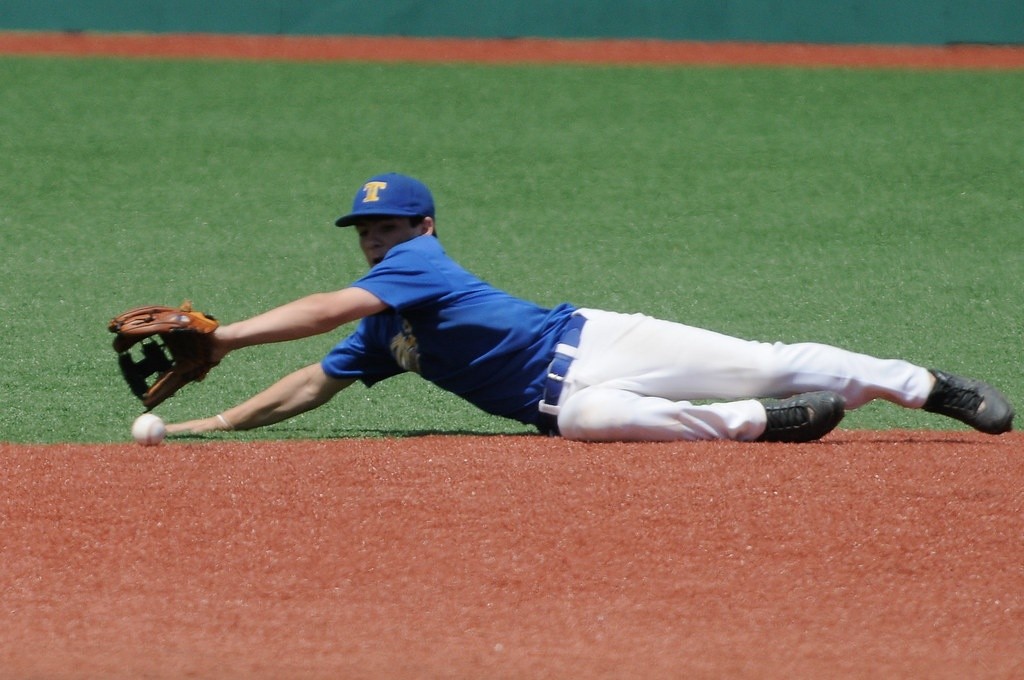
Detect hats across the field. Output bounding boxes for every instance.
[335,172,435,227]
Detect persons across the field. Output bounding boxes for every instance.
[106,172,1016,442]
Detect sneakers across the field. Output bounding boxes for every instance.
[753,390,845,440]
[922,369,1014,434]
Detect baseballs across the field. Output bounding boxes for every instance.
[131,414,166,446]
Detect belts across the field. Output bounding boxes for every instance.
[541,313,587,436]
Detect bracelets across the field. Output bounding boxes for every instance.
[216,415,231,430]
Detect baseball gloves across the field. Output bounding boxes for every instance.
[108,299,220,409]
